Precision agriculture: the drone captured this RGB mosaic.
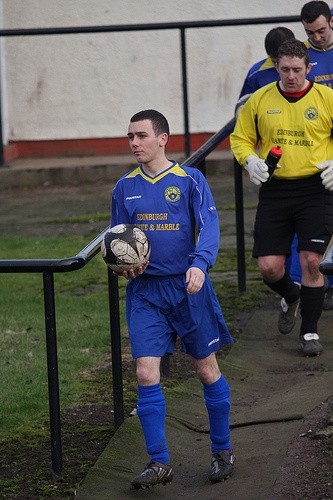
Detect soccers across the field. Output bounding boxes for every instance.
[100,223,151,274]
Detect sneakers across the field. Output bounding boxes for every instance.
[278,282,302,334]
[296,332,323,358]
[209,448,237,482]
[132,461,174,490]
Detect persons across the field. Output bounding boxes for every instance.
[230,40,333,356]
[111,110,236,490]
[235,27,296,120]
[300,0,333,89]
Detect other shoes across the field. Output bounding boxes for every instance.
[323,287,333,310]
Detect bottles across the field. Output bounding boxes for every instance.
[259,145,282,189]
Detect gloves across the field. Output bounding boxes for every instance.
[317,160,333,191]
[244,155,280,185]
